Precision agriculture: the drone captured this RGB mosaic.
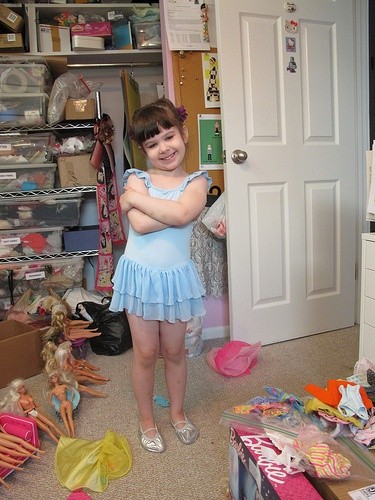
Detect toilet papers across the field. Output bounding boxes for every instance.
[1,67,28,94]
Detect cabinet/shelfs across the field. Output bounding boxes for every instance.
[359,232,375,387]
[0,0,163,64]
[0,92,112,318]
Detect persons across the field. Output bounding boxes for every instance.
[40,312,110,398]
[41,370,75,438]
[109,98,212,454]
[0,377,66,445]
[0,426,45,489]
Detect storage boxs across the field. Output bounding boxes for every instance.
[0,9,160,391]
[229,423,324,500]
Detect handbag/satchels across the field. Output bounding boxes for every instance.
[205,340,262,378]
[201,190,227,239]
[5,282,132,356]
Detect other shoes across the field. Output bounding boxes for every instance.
[138,420,166,454]
[170,411,198,444]
